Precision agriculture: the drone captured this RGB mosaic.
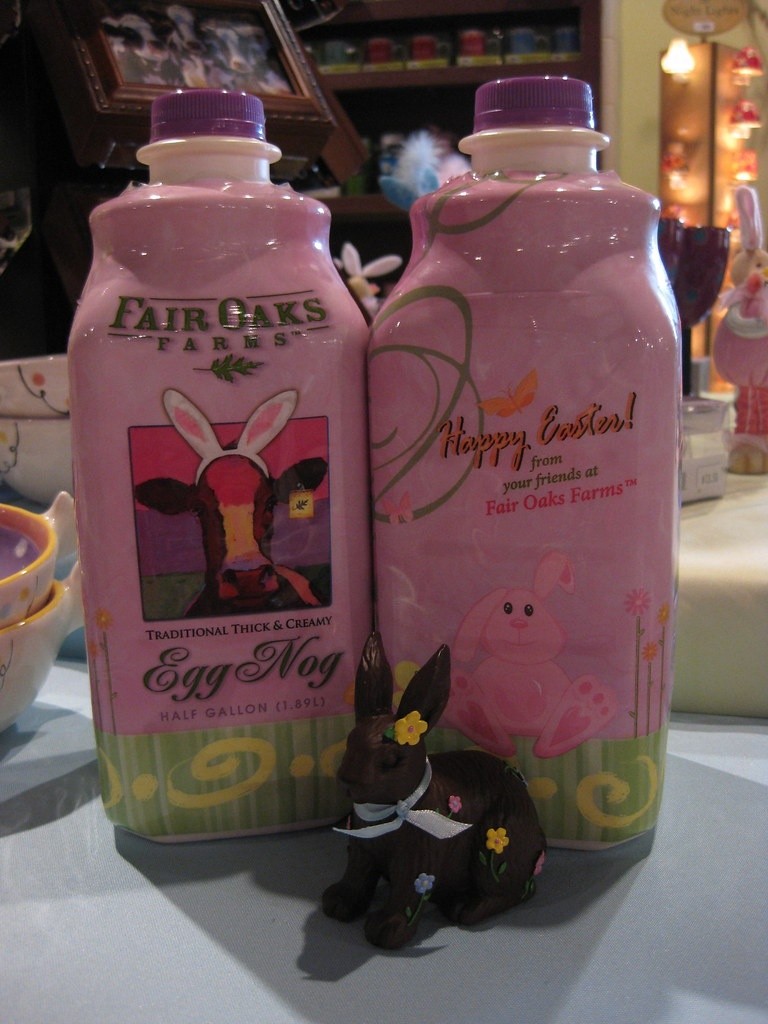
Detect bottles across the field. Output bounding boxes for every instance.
[68,89,368,845]
[367,76,680,849]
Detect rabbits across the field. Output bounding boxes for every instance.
[323,631,546,952]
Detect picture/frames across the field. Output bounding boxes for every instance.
[27,0,338,170]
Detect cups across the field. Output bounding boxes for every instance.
[1,354,69,417]
[0,563,84,733]
[0,414,73,504]
[1,493,76,626]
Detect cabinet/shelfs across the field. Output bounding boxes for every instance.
[322,0,602,225]
